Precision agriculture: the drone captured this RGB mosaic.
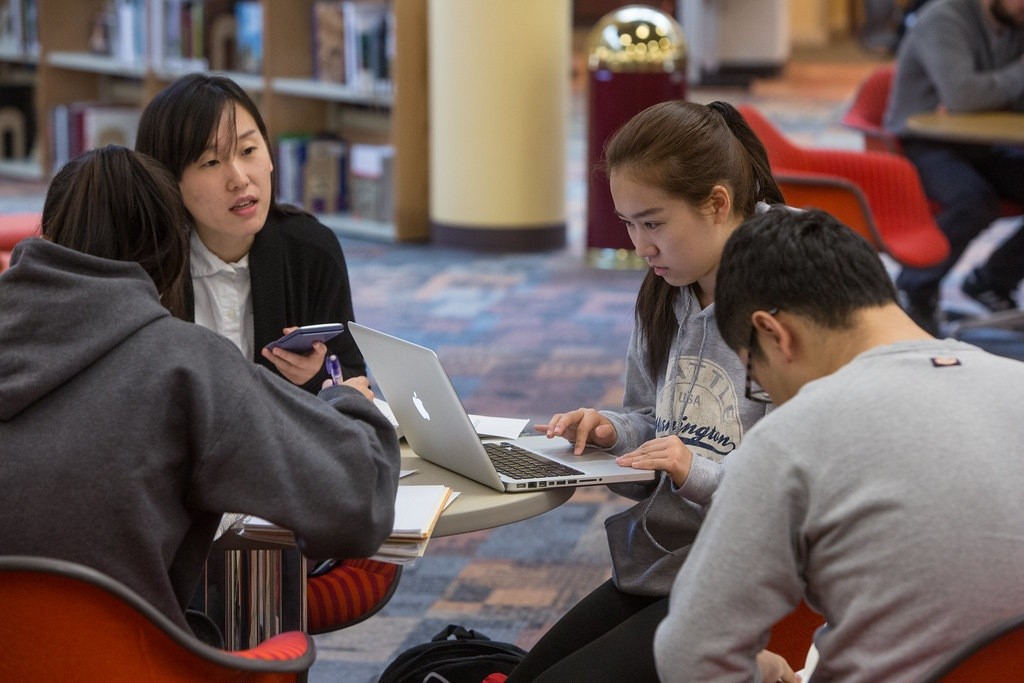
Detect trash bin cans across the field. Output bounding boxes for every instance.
[583,5,687,272]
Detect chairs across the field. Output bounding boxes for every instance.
[0,553,315,683]
[736,103,952,268]
[845,65,1019,219]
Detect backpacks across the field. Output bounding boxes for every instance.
[378,622,530,683]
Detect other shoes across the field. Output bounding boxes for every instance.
[903,289,942,341]
[962,275,1012,311]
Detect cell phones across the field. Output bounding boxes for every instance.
[261,324,344,356]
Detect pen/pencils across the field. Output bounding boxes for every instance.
[325,354,344,386]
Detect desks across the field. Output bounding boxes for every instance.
[907,112,1024,336]
[226,437,576,651]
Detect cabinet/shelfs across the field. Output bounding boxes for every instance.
[0,0,430,245]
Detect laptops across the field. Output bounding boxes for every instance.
[347,320,656,493]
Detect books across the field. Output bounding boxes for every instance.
[1,0,394,229]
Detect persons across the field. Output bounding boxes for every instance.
[504,100,783,683]
[133,73,381,396]
[0,147,398,647]
[652,206,1024,682]
[886,0,1023,339]
